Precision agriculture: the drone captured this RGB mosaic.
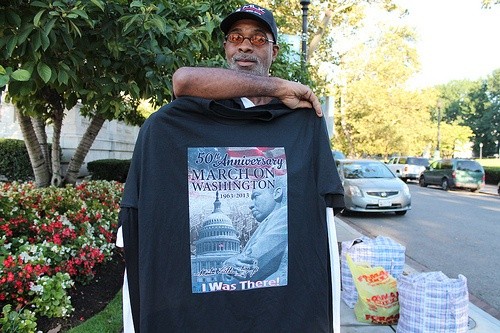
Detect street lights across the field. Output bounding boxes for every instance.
[479,142,484,160]
[435,102,443,160]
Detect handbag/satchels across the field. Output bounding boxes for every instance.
[341,232,469,333]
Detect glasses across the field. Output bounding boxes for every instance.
[225,32,274,46]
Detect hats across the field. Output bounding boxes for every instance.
[220,3,277,45]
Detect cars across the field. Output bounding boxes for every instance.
[334,159,412,216]
[384,156,434,183]
[418,158,485,191]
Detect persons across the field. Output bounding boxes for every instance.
[172,3,324,117]
